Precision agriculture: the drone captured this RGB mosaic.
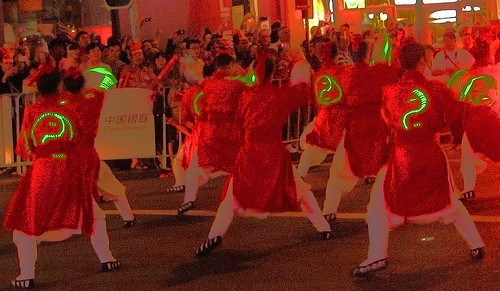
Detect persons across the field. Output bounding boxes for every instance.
[0,17,500,276]
[1,72,122,288]
[196,49,336,257]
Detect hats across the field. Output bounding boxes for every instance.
[127,40,142,54]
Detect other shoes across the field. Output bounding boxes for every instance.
[178,201,195,215]
[124,220,135,227]
[321,231,333,241]
[364,177,375,183]
[166,185,184,192]
[160,172,174,180]
[323,213,336,221]
[102,260,121,271]
[352,258,387,277]
[193,235,222,257]
[1,277,38,290]
[457,190,475,201]
[470,248,483,260]
